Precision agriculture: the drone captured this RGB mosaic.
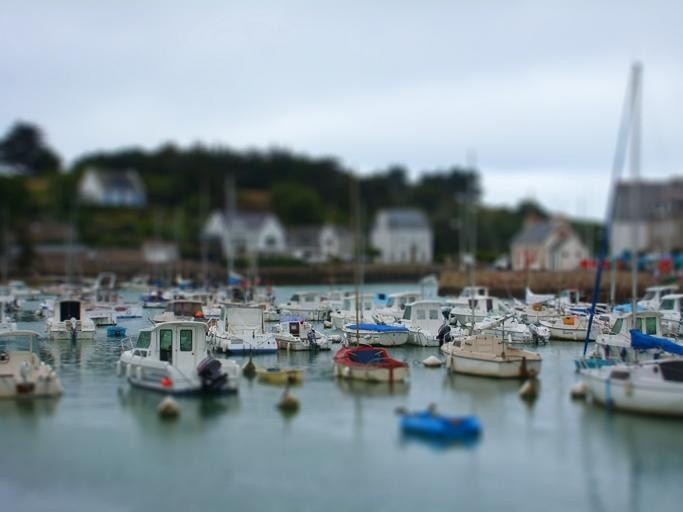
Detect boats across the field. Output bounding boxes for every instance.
[113,317,242,395]
[0,329,67,401]
[440,336,541,380]
[401,409,482,441]
[255,368,301,387]
[595,329,681,364]
[332,344,406,382]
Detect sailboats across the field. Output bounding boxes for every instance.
[575,63,682,415]
